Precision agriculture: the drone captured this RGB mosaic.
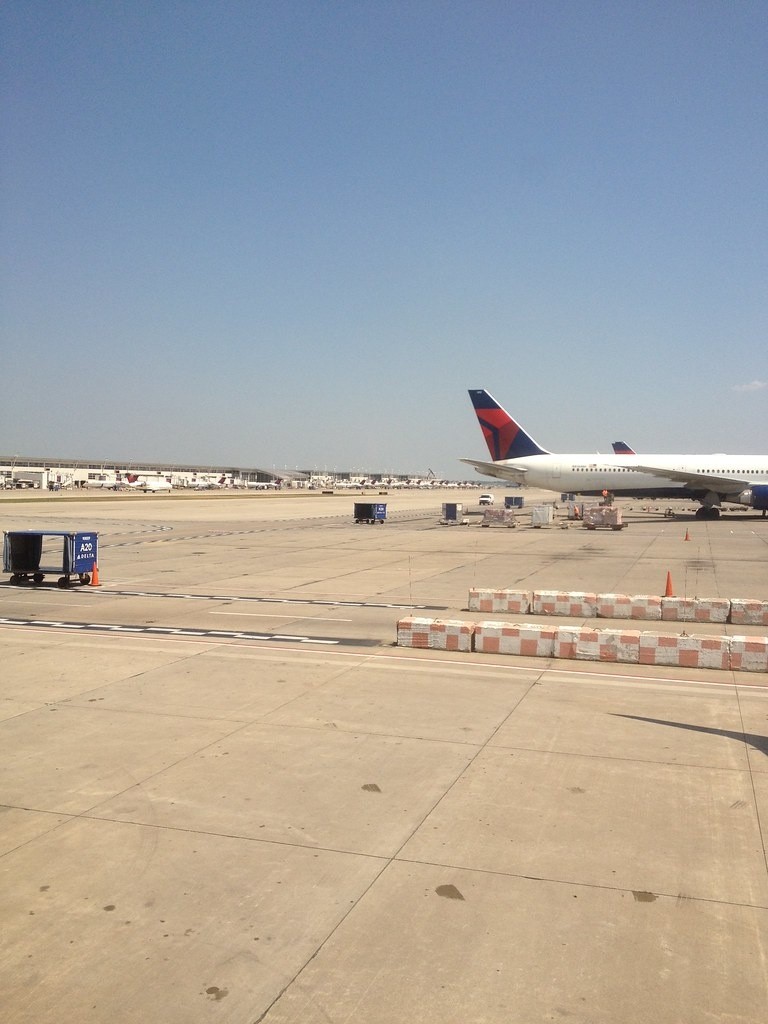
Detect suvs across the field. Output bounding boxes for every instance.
[479,494,494,506]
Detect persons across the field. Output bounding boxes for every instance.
[602,489,607,500]
[574,505,579,520]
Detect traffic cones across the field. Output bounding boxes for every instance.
[684,528,692,542]
[662,571,678,599]
[87,562,102,586]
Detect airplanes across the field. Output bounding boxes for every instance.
[244,480,478,490]
[458,389,768,523]
[62,478,173,494]
